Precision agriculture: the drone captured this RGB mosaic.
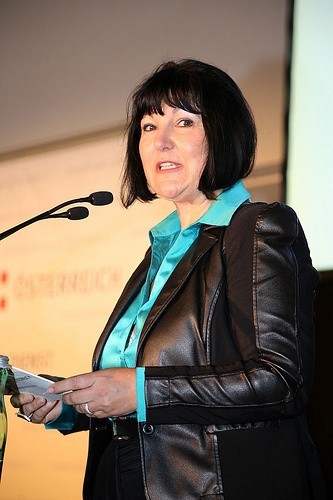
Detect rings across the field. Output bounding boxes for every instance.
[85,402,93,415]
[17,412,33,422]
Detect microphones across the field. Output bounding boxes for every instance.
[0,192,113,241]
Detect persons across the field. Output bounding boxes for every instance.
[9,58,333,500]
